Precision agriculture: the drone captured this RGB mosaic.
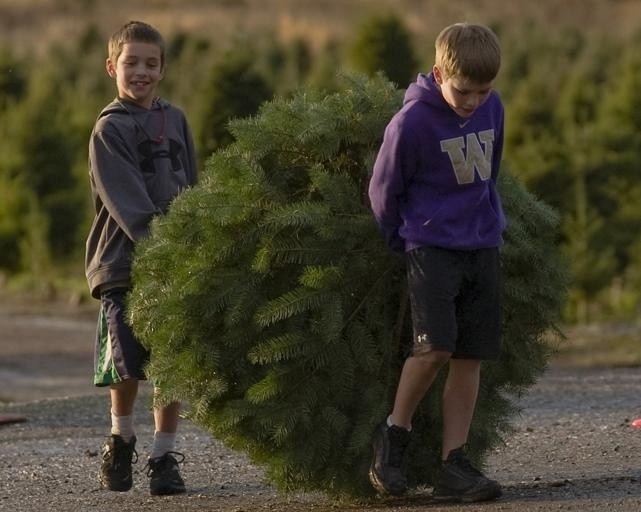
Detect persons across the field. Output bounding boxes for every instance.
[371,21,505,502]
[83,20,199,496]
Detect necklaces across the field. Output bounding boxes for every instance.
[115,97,166,143]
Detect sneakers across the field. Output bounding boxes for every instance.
[141,451,186,495]
[97,433,138,491]
[432,443,502,503]
[369,416,412,506]
